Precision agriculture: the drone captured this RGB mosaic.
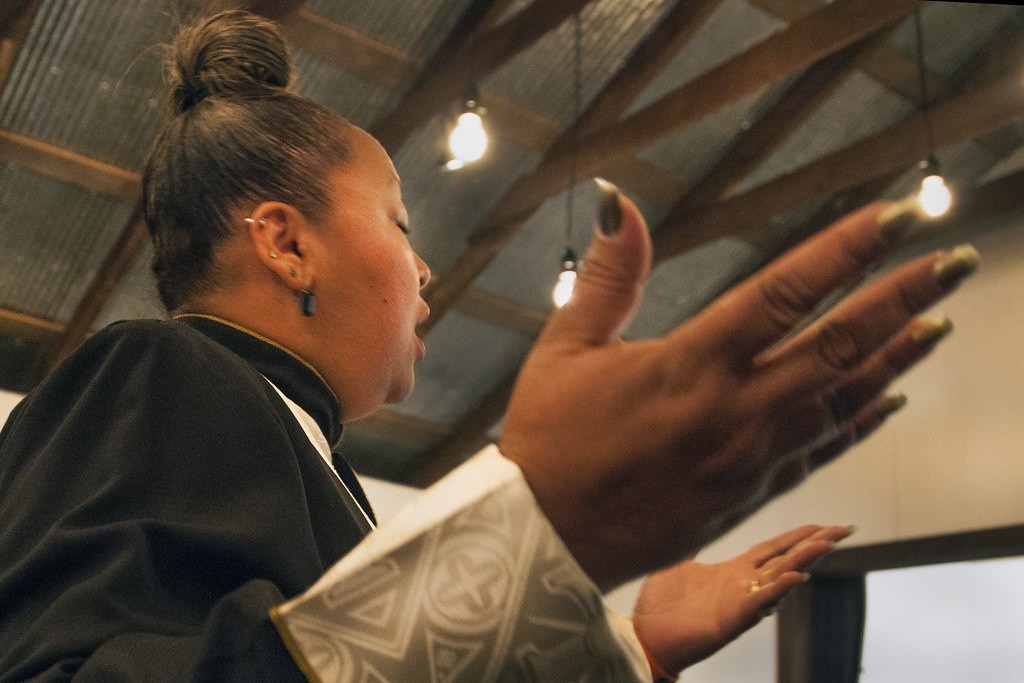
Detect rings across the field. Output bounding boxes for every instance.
[748,579,760,594]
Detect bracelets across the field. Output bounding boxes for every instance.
[630,616,679,683]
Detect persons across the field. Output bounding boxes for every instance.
[0,8,980,683]
